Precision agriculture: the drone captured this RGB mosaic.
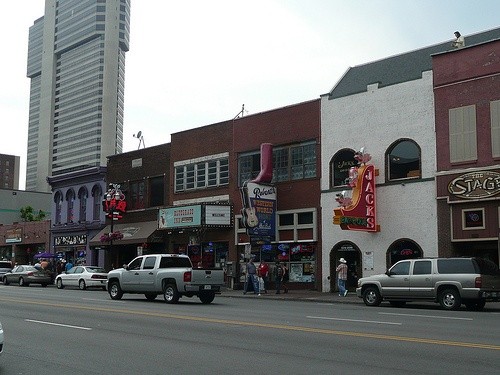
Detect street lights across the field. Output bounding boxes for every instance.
[105,188,121,270]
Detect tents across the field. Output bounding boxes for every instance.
[34,252,57,258]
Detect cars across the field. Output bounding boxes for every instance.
[0,261,52,287]
[54,266,110,290]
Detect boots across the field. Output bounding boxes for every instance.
[284,288,289,294]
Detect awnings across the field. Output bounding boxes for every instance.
[89,221,159,250]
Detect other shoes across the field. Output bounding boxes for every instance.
[343,290,349,297]
[338,294,340,297]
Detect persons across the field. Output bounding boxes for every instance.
[243,259,259,294]
[273,261,289,294]
[258,260,269,294]
[198,262,203,268]
[336,258,348,296]
[37,259,48,268]
[56,260,73,274]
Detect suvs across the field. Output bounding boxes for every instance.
[356,257,483,310]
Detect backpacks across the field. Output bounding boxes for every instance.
[275,265,283,277]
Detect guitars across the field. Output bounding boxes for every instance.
[237,187,260,228]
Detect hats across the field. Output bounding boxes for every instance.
[61,259,66,262]
[338,258,347,264]
[43,258,46,261]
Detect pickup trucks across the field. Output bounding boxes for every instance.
[106,253,224,304]
